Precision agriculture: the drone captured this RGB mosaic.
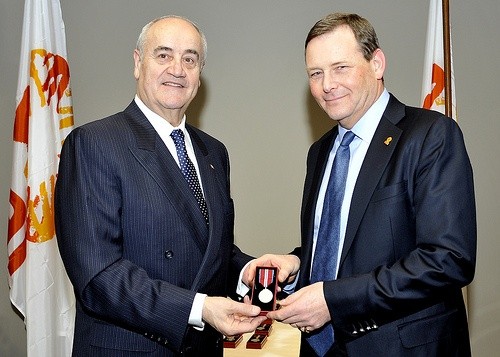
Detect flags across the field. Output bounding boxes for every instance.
[418,0,469,322]
[5,0,77,357]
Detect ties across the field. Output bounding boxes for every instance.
[171,129,210,227]
[305,130,355,357]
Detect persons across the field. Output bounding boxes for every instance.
[54,14,267,357]
[244,12,477,357]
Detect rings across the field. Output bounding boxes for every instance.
[299,325,307,333]
